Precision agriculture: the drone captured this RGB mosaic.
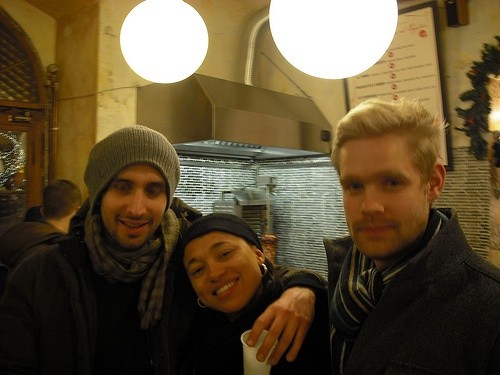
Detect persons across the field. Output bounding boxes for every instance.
[322,97,496,374]
[174,213,331,375]
[0,125,329,374]
[0,179,84,297]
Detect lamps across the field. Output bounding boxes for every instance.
[120,0,209,84]
[268,0,399,80]
[485,74,499,132]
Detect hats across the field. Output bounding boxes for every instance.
[84,125,180,216]
[178,213,263,260]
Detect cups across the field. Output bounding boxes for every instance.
[240,328,280,375]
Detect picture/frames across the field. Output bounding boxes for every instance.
[343,0,453,171]
